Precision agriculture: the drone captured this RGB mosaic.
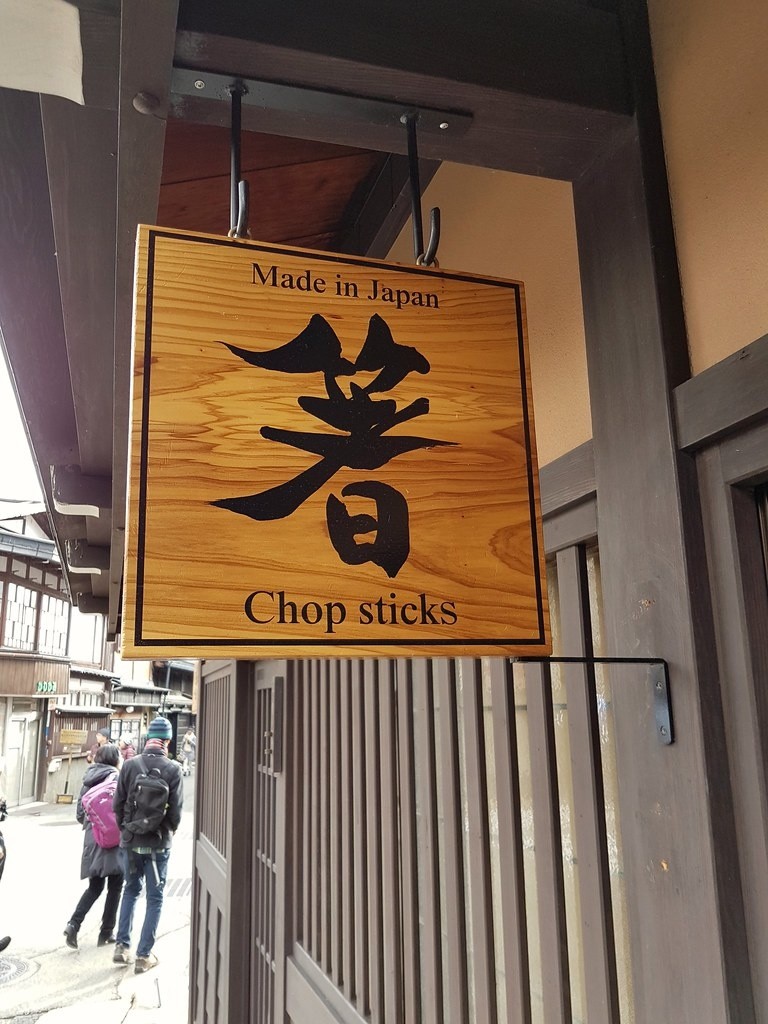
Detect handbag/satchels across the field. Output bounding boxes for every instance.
[184,741,191,752]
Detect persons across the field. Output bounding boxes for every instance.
[181,727,196,776]
[64,728,136,948]
[113,717,183,973]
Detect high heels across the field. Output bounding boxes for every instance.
[64,923,78,949]
[97,932,117,945]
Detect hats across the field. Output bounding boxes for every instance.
[120,733,134,745]
[98,728,110,738]
[147,717,172,739]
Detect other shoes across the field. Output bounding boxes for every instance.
[189,771,191,775]
[134,951,160,972]
[0,936,11,951]
[112,945,134,964]
[183,772,186,776]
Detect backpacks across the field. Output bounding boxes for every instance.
[121,754,181,836]
[82,773,122,849]
